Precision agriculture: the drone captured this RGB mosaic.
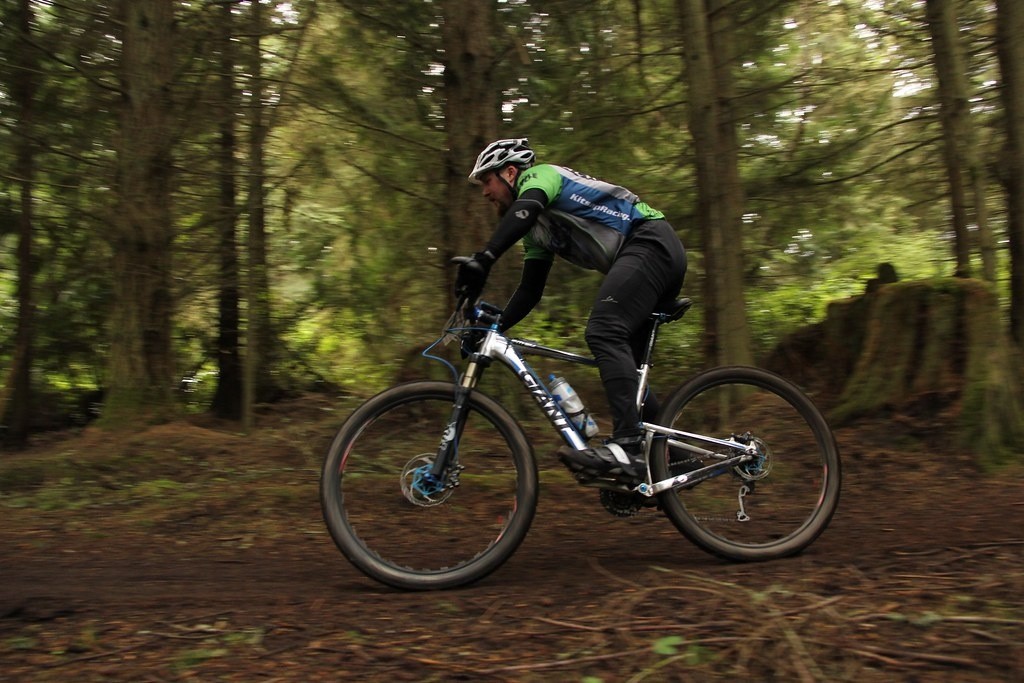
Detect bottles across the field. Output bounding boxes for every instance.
[547,374,598,437]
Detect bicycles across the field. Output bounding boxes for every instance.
[319,252,841,591]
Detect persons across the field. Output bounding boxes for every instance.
[454,137,705,485]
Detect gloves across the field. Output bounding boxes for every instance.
[453,253,493,302]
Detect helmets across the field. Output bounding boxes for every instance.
[468,139,535,185]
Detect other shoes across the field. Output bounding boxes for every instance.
[556,443,647,474]
